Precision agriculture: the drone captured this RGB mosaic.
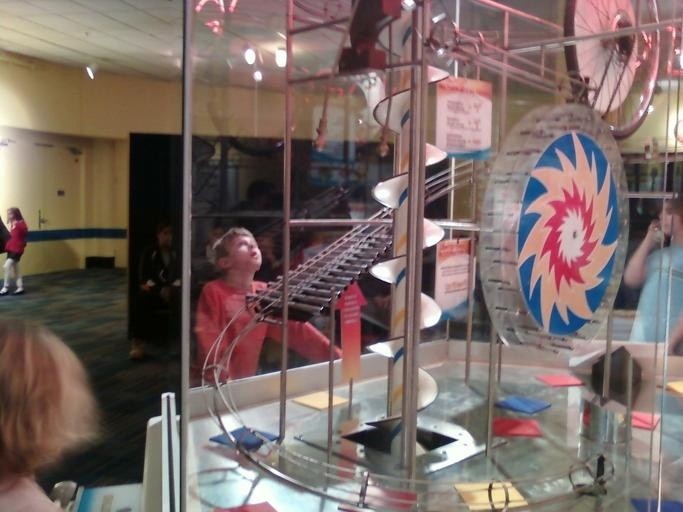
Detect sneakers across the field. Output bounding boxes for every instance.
[0,289,8,295]
[12,290,24,295]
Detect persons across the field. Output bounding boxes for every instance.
[189,178,390,337]
[0,207,28,295]
[128,222,180,362]
[0,319,102,511]
[189,226,342,389]
[624,198,683,355]
